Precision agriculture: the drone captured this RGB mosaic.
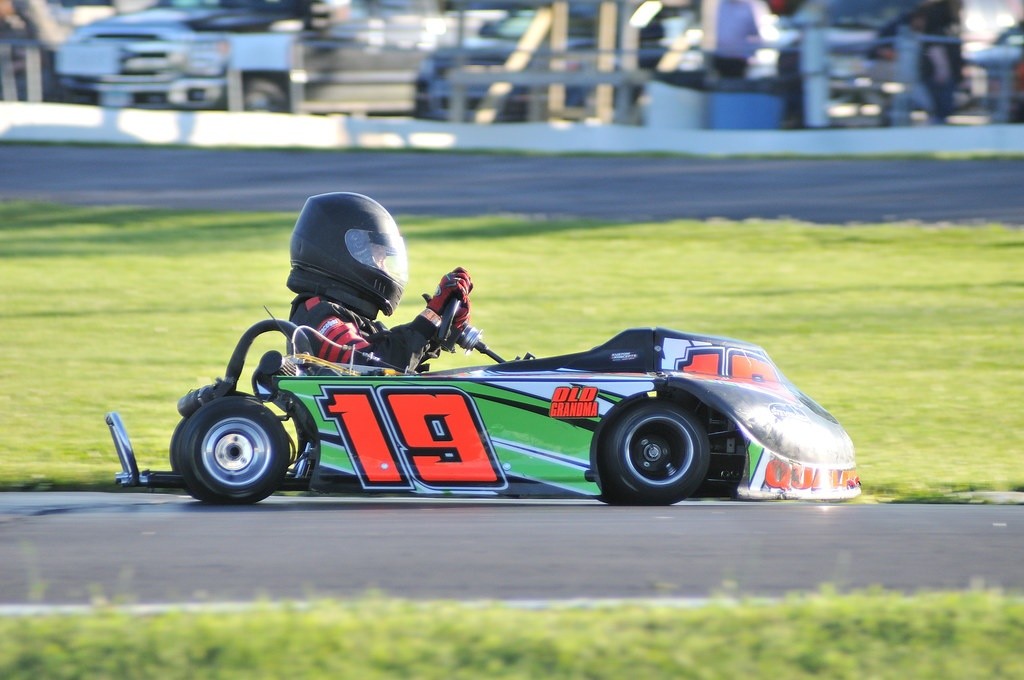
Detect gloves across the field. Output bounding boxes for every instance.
[420,267,474,336]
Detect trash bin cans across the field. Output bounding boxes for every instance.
[706,76,793,130]
[639,70,710,128]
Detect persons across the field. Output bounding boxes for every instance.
[693,0,771,90]
[909,16,972,125]
[286,191,474,373]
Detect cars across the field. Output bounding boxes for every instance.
[414,0,773,122]
[777,0,1024,118]
[55,0,477,113]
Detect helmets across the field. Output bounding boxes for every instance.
[289,192,409,318]
